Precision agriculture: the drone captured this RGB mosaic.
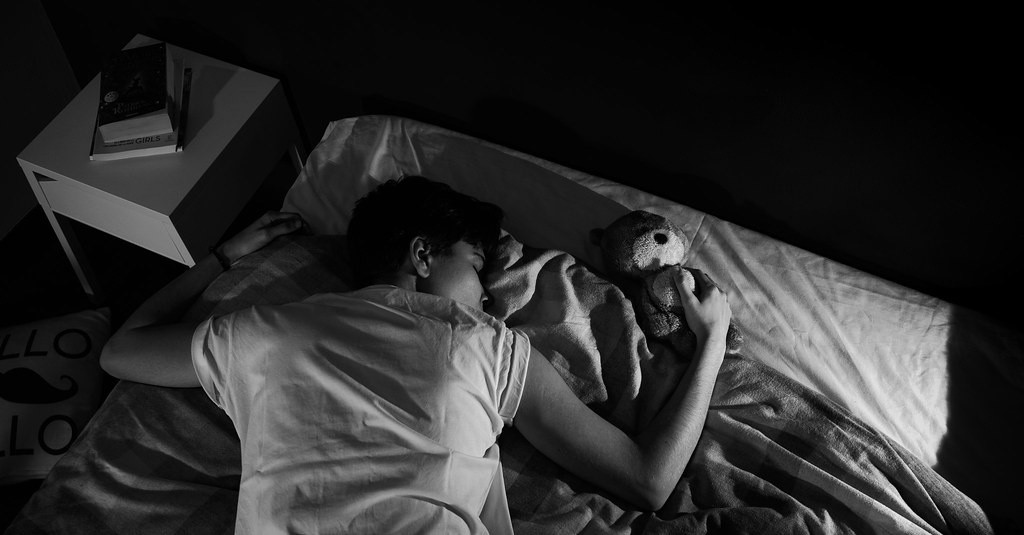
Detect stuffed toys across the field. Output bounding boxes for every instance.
[589,210,744,361]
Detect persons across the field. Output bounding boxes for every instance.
[99,175,733,535]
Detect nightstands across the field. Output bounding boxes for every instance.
[15,33,302,296]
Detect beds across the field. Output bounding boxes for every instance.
[0,118,1024,535]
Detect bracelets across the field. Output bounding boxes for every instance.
[208,244,229,272]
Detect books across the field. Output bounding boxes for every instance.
[88,41,191,164]
